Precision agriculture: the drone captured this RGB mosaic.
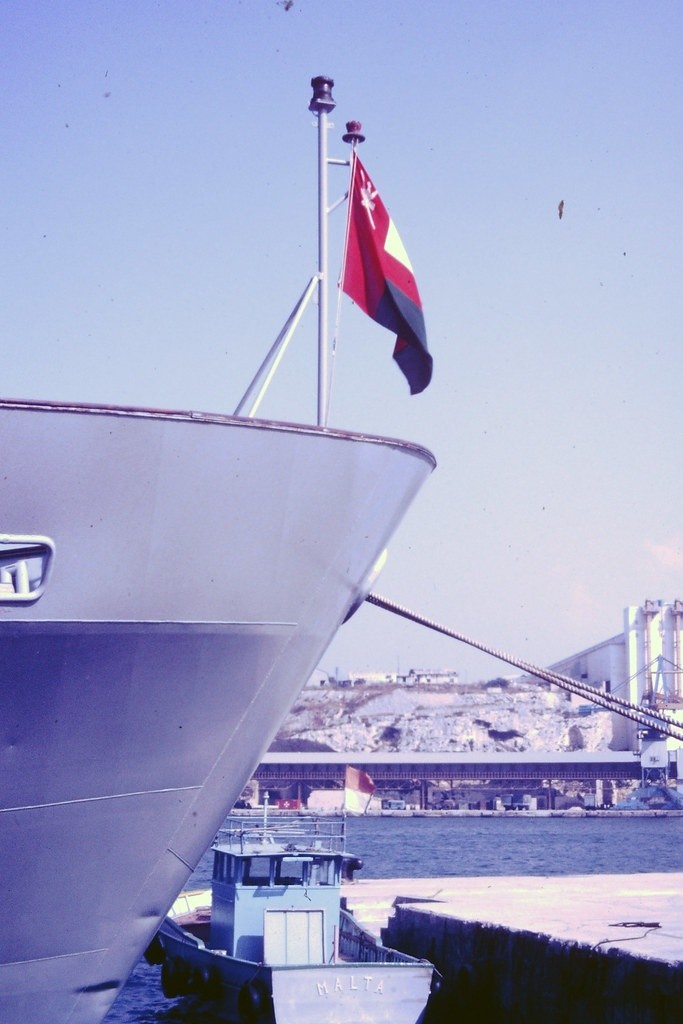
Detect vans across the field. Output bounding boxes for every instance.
[384,800,405,809]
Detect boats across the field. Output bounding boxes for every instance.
[158,790,435,1024]
[0,75,436,1024]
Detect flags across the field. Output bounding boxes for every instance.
[344,765,376,815]
[337,149,433,395]
